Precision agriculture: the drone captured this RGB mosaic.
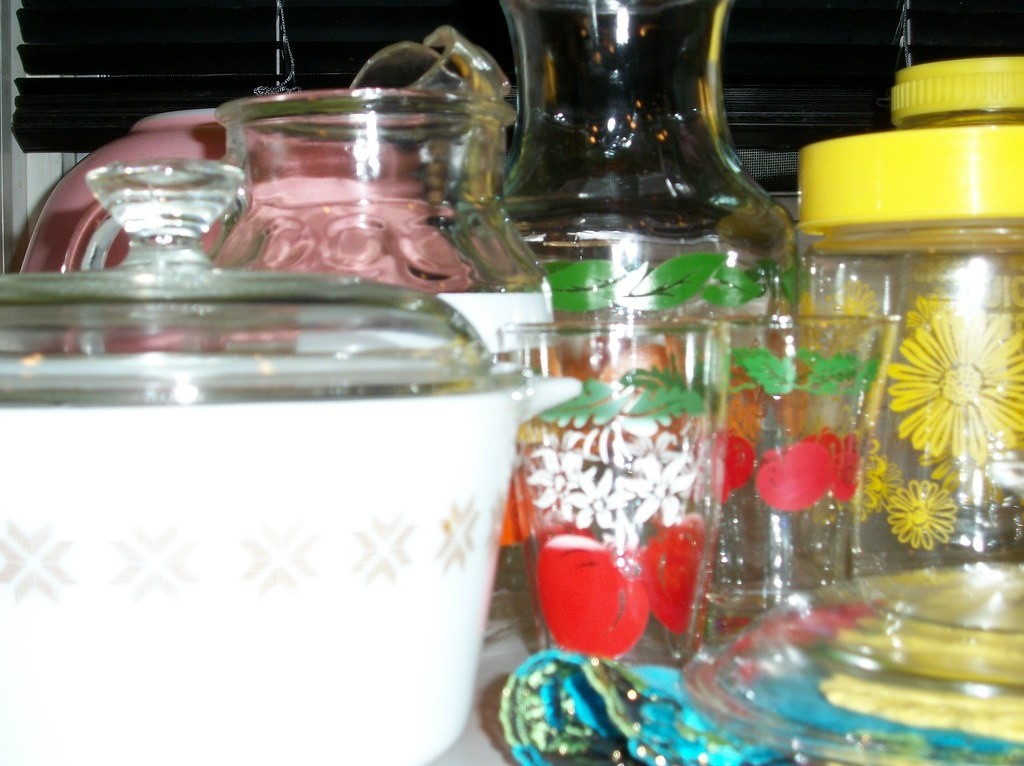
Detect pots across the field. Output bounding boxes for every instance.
[0,161,580,766]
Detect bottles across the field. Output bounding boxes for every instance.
[214,0,798,764]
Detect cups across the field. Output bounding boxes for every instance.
[498,219,898,665]
